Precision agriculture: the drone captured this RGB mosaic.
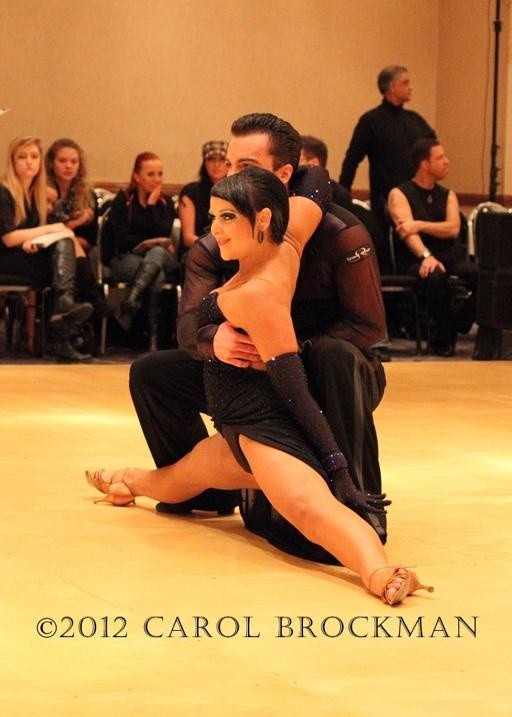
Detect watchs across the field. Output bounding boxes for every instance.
[416,249,432,262]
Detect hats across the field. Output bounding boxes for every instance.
[203,141,228,158]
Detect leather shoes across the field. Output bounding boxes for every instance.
[156,489,234,515]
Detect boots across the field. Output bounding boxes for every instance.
[122,262,161,314]
[43,239,93,361]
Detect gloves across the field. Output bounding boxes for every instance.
[322,452,391,514]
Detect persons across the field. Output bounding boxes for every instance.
[339,67,436,217]
[176,139,229,284]
[298,136,353,212]
[0,135,117,363]
[385,136,504,361]
[84,164,434,605]
[129,112,386,567]
[99,152,183,352]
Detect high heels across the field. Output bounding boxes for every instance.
[86,469,135,506]
[369,566,433,607]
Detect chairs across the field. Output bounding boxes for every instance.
[1,186,512,358]
